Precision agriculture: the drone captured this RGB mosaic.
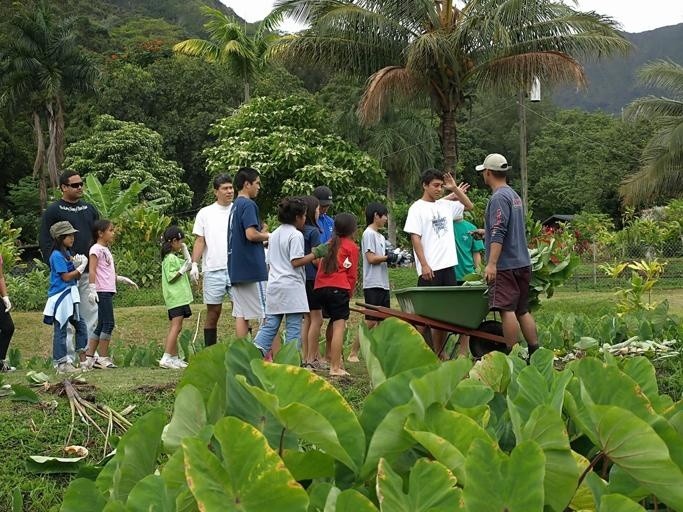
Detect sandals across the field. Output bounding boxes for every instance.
[299,361,319,375]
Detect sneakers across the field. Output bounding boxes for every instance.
[173,356,191,368]
[93,356,119,371]
[0,360,17,377]
[79,357,95,373]
[158,356,179,370]
[78,358,104,369]
[54,359,82,373]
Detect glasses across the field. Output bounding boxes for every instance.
[60,178,85,189]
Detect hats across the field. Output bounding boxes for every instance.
[311,185,335,209]
[49,219,80,240]
[474,152,514,173]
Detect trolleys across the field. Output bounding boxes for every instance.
[349,285,509,357]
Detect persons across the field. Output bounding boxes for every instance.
[85,219,139,370]
[252,196,330,359]
[189,173,253,348]
[346,202,411,363]
[438,181,486,363]
[38,169,101,372]
[312,185,345,364]
[403,167,474,362]
[313,212,359,376]
[300,195,331,373]
[0,241,17,373]
[465,152,540,366]
[43,221,88,375]
[158,225,193,370]
[227,167,281,361]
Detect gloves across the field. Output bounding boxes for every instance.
[71,253,81,267]
[1,295,14,313]
[310,242,331,260]
[116,275,140,293]
[179,241,193,261]
[177,259,194,276]
[191,259,200,286]
[87,282,101,307]
[391,251,412,268]
[75,252,89,276]
[387,246,405,266]
